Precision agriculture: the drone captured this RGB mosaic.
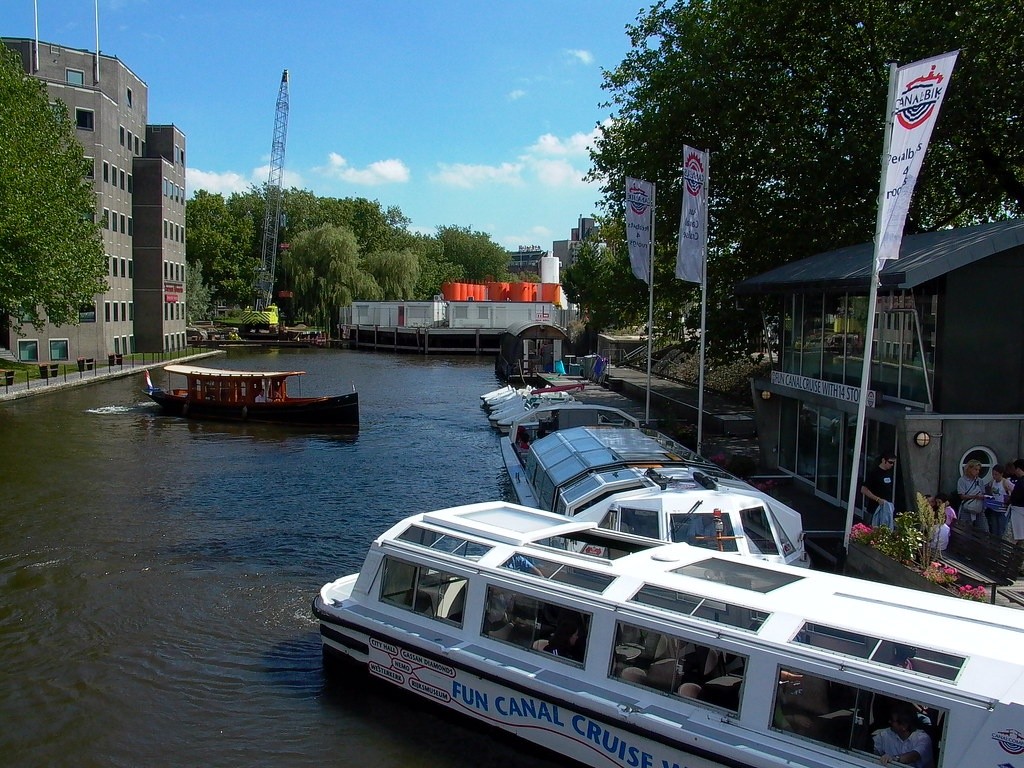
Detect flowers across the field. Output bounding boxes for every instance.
[856,512,990,604]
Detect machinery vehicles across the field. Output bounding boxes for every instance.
[224,69,290,335]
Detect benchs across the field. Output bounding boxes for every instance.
[892,518,1024,607]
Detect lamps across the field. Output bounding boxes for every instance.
[760,390,772,401]
[914,431,931,447]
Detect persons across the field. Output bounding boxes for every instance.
[861,451,1024,562]
[255,389,270,402]
[481,514,934,768]
[516,426,531,450]
[540,343,552,374]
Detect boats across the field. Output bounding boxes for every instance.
[308,498,1024,768]
[494,317,569,385]
[141,364,361,435]
[479,378,635,433]
[498,395,811,591]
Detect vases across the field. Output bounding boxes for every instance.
[842,540,968,599]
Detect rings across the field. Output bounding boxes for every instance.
[807,723,810,726]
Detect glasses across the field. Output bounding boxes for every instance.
[887,461,895,465]
[889,714,899,723]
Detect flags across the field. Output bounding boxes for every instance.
[145,368,152,389]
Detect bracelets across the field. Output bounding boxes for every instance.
[792,715,796,725]
[876,497,881,501]
[920,710,924,713]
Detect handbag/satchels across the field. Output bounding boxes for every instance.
[957,504,976,526]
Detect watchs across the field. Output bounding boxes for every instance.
[894,755,901,762]
